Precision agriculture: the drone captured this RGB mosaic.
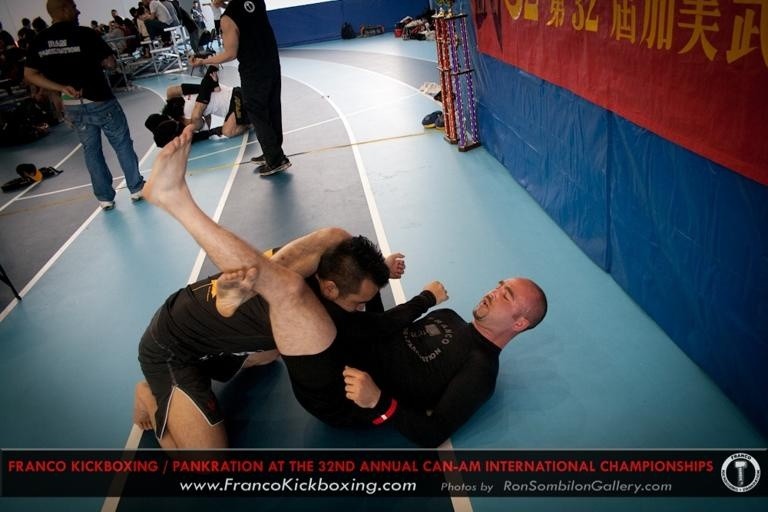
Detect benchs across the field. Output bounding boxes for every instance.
[0,78,37,127]
[103,19,195,94]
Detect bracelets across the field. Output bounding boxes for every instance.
[370,399,397,426]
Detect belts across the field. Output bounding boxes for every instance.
[61,98,94,106]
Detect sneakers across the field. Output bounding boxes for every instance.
[130,189,144,202]
[99,199,116,211]
[435,114,445,131]
[419,81,456,103]
[421,110,443,129]
[258,154,293,176]
[250,153,266,165]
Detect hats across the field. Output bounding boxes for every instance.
[15,161,44,182]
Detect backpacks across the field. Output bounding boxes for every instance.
[341,20,357,40]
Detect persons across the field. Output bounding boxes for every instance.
[142,123,548,446]
[187,0,292,176]
[135,234,453,486]
[22,1,146,210]
[0,0,253,149]
